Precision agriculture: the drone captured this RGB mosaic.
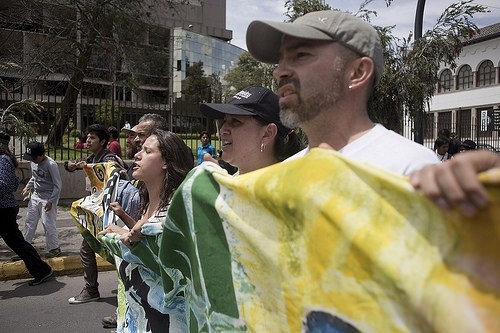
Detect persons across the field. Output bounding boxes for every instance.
[160,86,303,230]
[11,141,64,260]
[197,131,216,165]
[246,10,443,182]
[0,131,54,286]
[410,129,500,219]
[64,124,123,304]
[101,113,194,328]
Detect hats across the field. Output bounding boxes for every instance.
[23,141,44,161]
[459,140,476,150]
[246,10,383,87]
[121,125,139,132]
[200,86,289,138]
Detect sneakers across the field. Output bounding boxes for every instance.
[45,247,62,257]
[28,268,54,286]
[68,286,100,303]
[11,254,22,260]
[102,312,117,327]
